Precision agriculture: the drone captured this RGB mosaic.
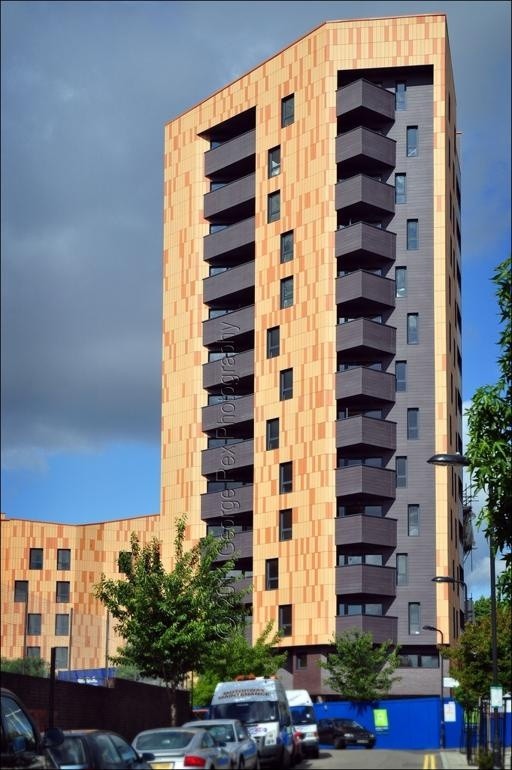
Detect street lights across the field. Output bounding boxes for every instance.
[422,625,445,747]
[427,454,499,753]
[432,577,472,766]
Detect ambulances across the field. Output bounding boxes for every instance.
[208,676,296,765]
[287,688,320,757]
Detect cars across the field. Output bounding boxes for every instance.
[317,717,376,749]
[132,728,231,770]
[1,688,154,769]
[182,719,261,770]
